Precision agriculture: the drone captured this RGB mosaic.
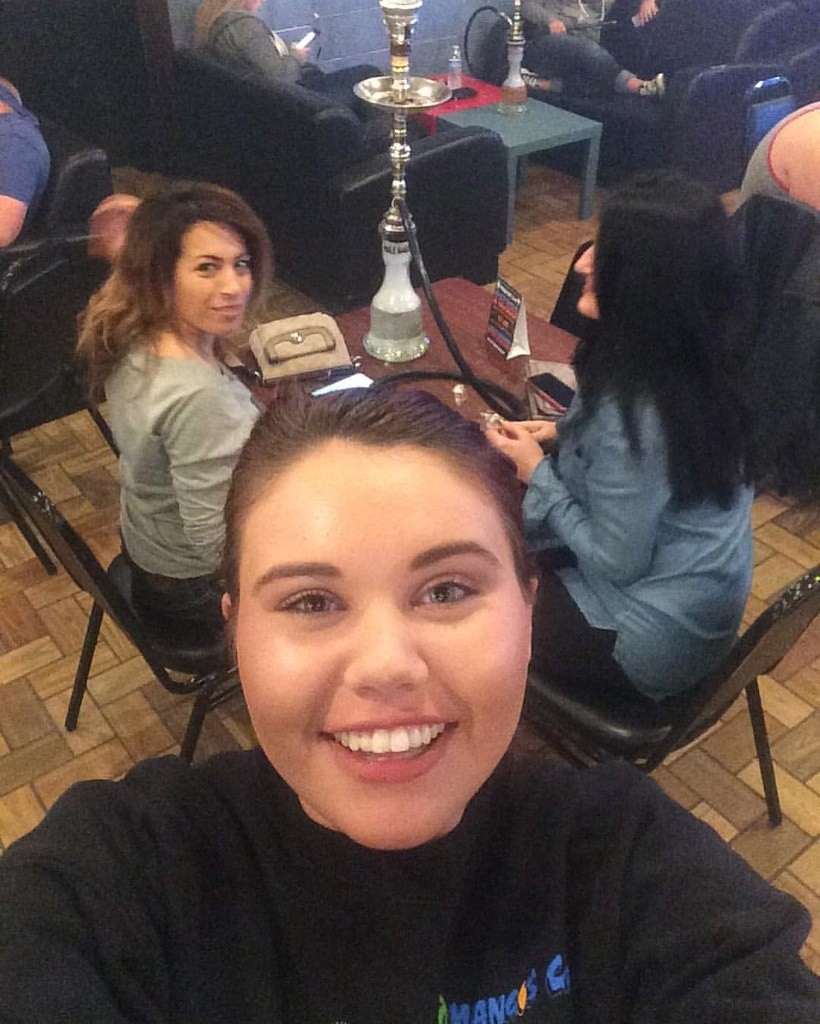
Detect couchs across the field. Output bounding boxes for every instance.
[177,47,509,315]
[1,113,114,433]
[527,0,820,185]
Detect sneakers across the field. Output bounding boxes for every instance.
[639,74,664,105]
[520,66,542,92]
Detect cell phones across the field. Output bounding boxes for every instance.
[295,28,320,48]
[309,373,373,397]
[631,12,641,28]
[528,371,576,412]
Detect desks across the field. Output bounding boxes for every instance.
[408,74,603,219]
[247,276,583,436]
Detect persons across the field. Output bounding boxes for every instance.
[485,168,756,723]
[738,101,820,214]
[76,174,264,636]
[191,0,384,121]
[0,392,820,1024]
[521,0,665,105]
[0,68,52,249]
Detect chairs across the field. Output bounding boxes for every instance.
[551,240,599,340]
[724,194,820,508]
[740,75,795,186]
[1,436,243,763]
[518,563,820,826]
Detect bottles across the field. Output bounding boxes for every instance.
[447,45,463,90]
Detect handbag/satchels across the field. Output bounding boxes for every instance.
[248,312,363,387]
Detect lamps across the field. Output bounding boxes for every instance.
[352,0,451,361]
[497,0,526,114]
